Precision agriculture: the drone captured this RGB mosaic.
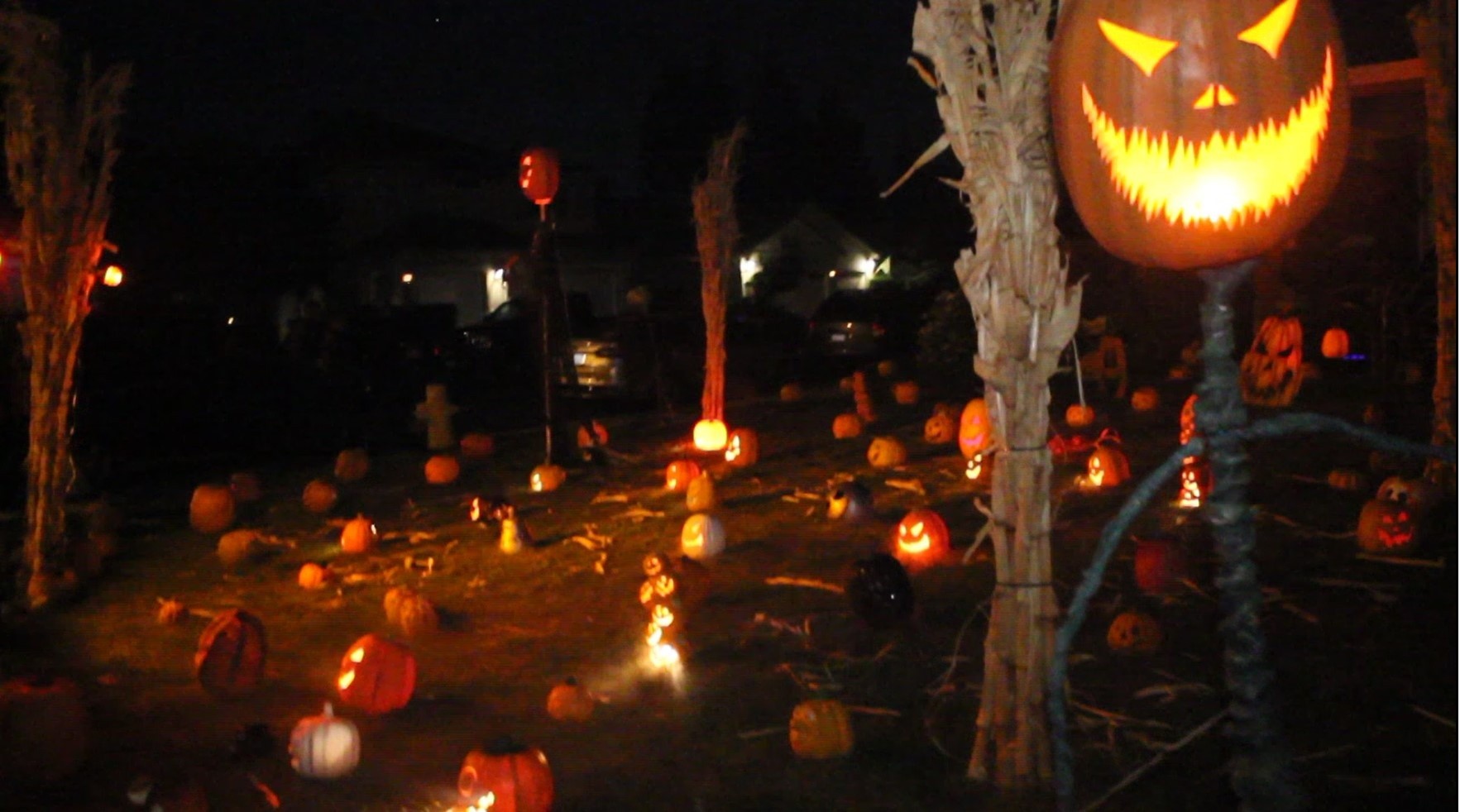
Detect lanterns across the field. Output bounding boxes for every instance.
[186,0,1425,812]
